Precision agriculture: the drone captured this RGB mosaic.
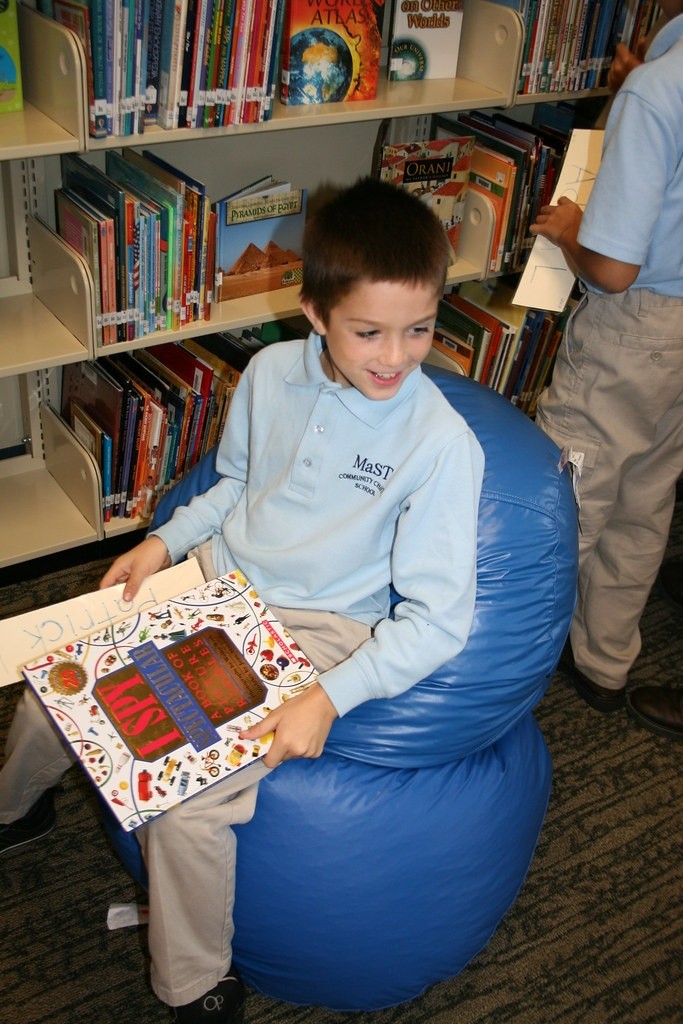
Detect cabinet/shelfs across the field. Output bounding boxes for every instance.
[1,0,681,574]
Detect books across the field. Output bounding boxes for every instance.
[0,0,25,113]
[19,568,319,831]
[489,0,663,95]
[37,0,464,139]
[60,320,308,521]
[53,147,308,346]
[431,275,579,411]
[379,98,598,272]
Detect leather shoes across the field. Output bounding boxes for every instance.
[628,686,683,742]
[557,651,627,711]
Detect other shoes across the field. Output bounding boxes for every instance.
[173,961,246,1024]
[0,788,55,854]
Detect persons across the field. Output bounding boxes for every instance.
[530,0,683,710]
[0,176,485,1024]
